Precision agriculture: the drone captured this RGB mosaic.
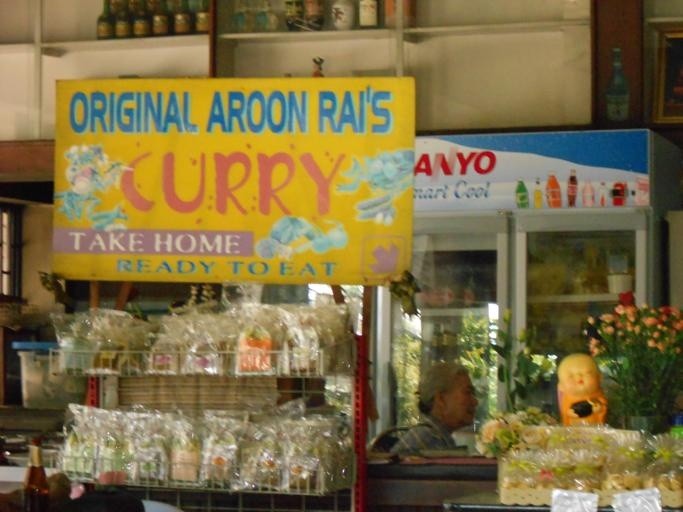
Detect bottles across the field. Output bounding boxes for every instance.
[516,168,626,208]
[24,436,49,512]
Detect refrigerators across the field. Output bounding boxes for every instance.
[375,130,652,436]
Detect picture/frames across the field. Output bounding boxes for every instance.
[648,21,683,126]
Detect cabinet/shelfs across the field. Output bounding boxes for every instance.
[45,336,357,496]
[3,1,593,142]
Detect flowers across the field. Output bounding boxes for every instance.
[474,288,680,457]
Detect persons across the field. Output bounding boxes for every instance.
[383,360,480,461]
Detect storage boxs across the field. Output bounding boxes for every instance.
[11,339,88,408]
